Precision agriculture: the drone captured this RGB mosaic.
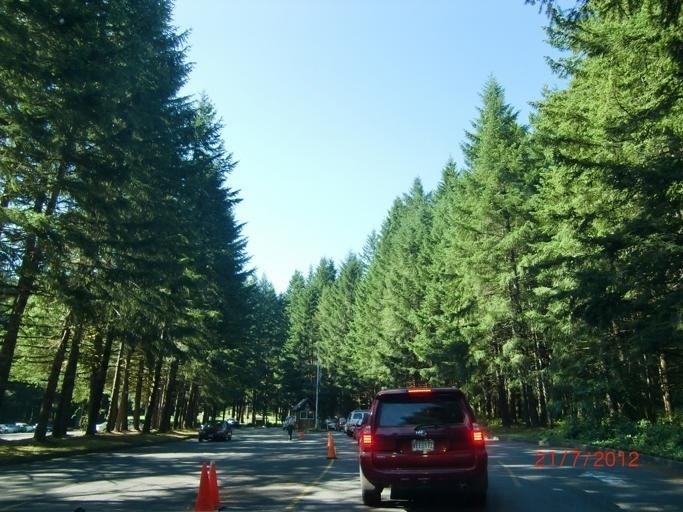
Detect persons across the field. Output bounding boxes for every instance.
[283,415,296,440]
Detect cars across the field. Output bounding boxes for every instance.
[198,420,232,441]
[0,420,54,433]
[323,385,488,506]
